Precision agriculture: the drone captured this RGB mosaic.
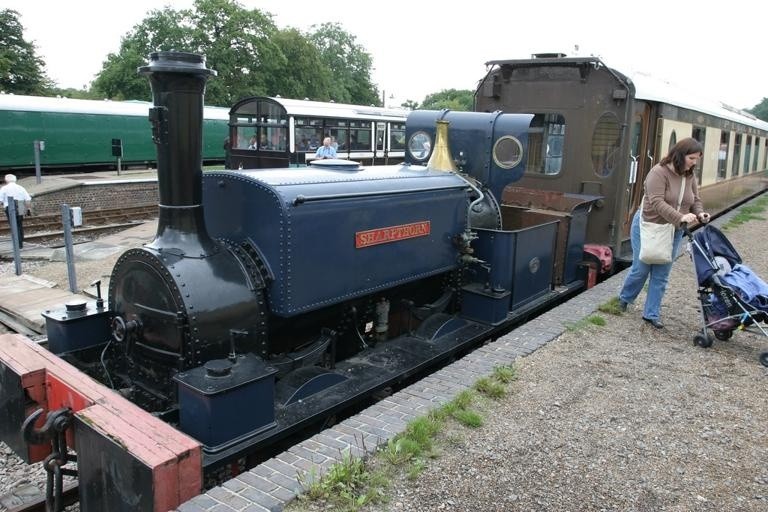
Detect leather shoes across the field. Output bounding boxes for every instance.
[642,316,663,329]
[619,297,627,312]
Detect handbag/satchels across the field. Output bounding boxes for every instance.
[639,221,675,265]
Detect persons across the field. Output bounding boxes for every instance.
[0,174,32,252]
[248,132,360,150]
[618,137,711,328]
[315,137,337,159]
